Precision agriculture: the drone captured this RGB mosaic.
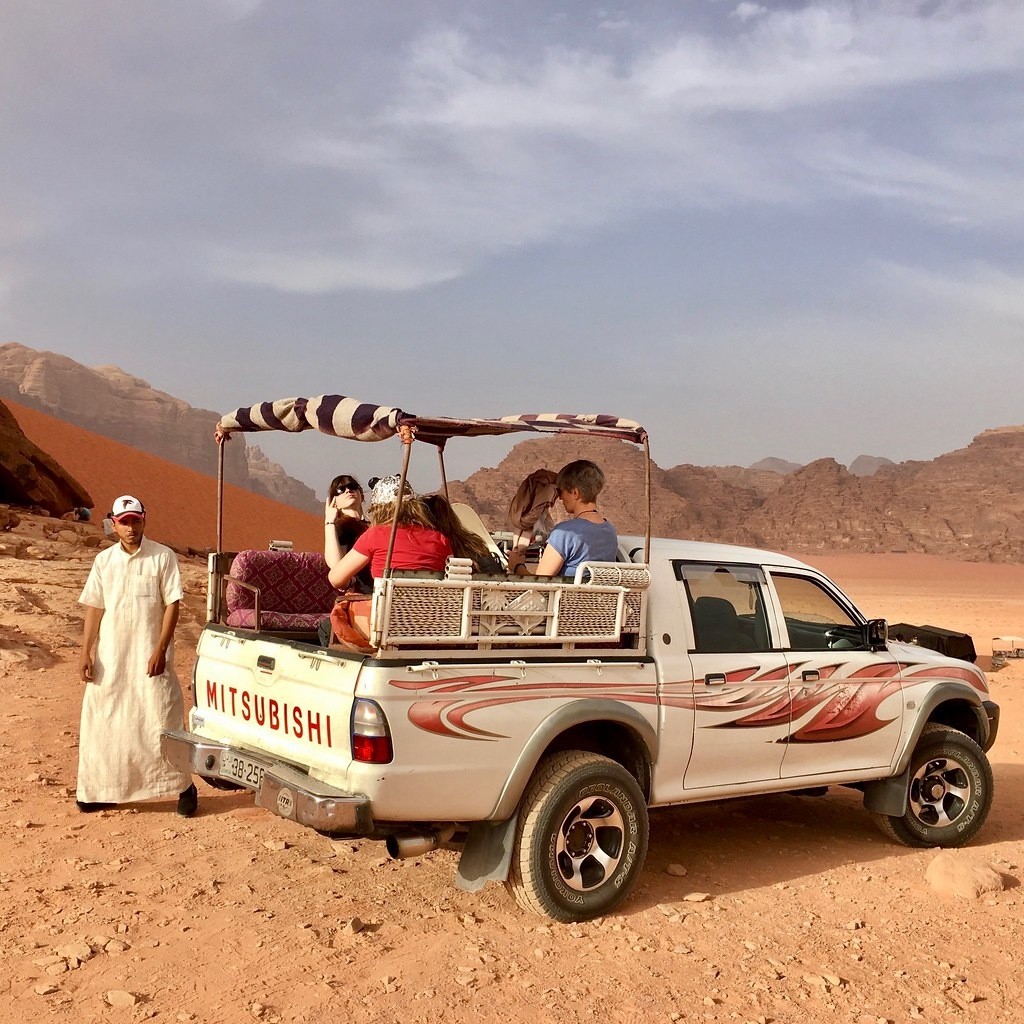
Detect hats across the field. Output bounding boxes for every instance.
[111,494,145,522]
[368,474,414,504]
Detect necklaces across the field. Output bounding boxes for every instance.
[575,509,600,520]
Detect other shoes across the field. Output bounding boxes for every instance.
[76,800,118,811]
[177,783,197,816]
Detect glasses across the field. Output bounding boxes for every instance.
[333,482,358,496]
[556,487,563,495]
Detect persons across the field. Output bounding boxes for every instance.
[317,475,455,647]
[76,494,200,819]
[415,495,508,573]
[504,459,619,576]
[322,474,376,595]
[101,512,117,542]
[73,504,92,522]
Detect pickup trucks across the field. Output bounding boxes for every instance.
[187,390,1004,925]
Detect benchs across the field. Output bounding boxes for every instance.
[210,550,346,641]
[330,560,652,658]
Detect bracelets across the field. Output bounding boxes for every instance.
[324,521,336,526]
[337,578,353,593]
[513,564,526,573]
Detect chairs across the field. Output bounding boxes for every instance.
[692,596,742,653]
